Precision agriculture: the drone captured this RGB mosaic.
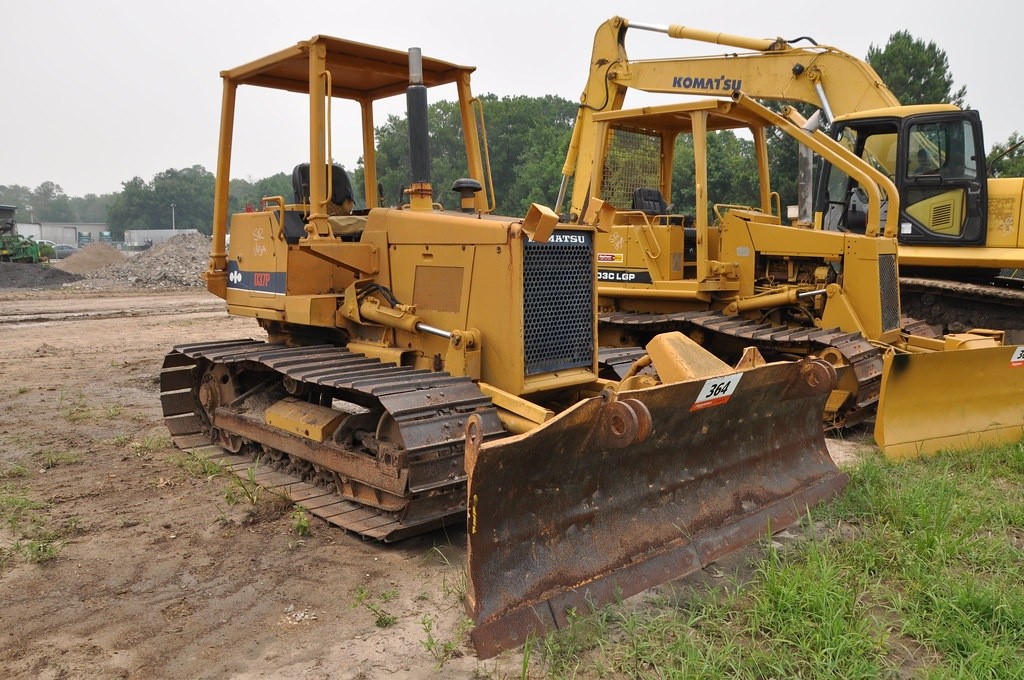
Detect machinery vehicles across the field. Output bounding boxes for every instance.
[1,204,55,264]
[553,16,1024,345]
[555,91,1024,462]
[156,34,853,659]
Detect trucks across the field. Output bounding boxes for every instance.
[78,231,95,248]
[100,230,112,244]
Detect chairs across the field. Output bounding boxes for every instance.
[293,161,355,222]
[633,187,670,226]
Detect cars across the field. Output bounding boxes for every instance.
[35,240,59,247]
[51,245,82,258]
